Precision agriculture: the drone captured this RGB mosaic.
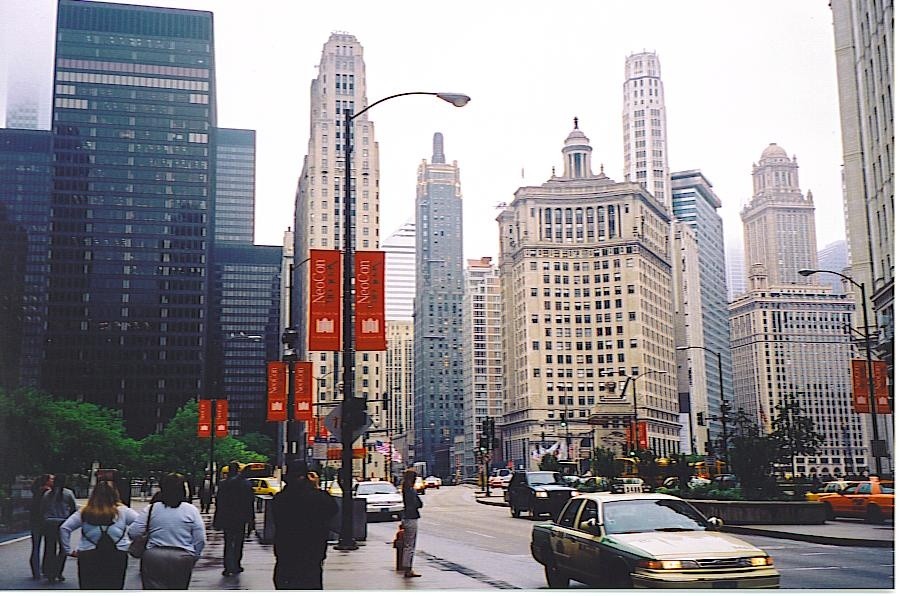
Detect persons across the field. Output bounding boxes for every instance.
[59,480,140,590]
[401,470,423,578]
[149,472,268,538]
[29,473,77,585]
[797,473,835,482]
[263,458,339,590]
[127,473,206,590]
[215,462,254,576]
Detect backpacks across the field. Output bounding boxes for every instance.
[81,519,125,563]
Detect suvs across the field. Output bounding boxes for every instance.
[508,470,579,521]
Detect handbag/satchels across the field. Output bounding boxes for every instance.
[128,503,154,557]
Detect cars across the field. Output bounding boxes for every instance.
[204,461,359,498]
[424,467,527,490]
[530,482,782,589]
[561,470,894,525]
[399,476,426,495]
[351,480,406,521]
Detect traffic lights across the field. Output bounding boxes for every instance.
[482,421,488,435]
[480,438,489,454]
[492,437,499,449]
[697,412,703,425]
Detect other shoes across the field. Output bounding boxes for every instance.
[404,573,421,578]
[49,573,64,583]
[223,566,243,576]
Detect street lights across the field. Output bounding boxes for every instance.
[601,370,667,452]
[675,345,728,473]
[336,91,471,546]
[797,268,883,479]
